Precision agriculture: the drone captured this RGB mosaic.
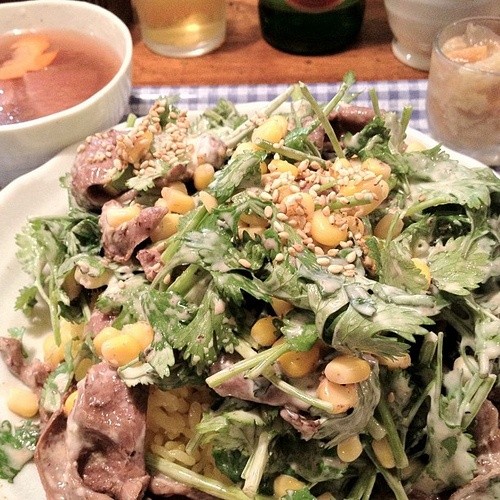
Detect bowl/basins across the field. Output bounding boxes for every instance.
[0,0,134,188]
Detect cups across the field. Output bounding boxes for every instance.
[426,16,500,167]
[384,0,500,72]
[134,0,227,58]
[257,0,365,56]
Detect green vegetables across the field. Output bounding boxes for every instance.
[0,68,500,500]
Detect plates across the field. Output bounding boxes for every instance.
[0,101,500,500]
